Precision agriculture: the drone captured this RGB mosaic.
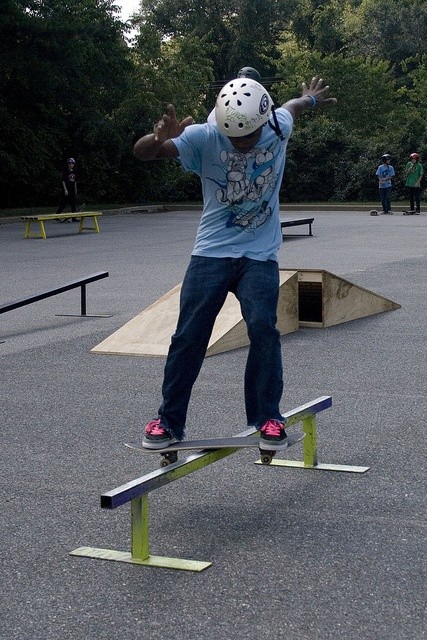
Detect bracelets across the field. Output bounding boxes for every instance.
[301,94,316,109]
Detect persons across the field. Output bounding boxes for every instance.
[376,154,396,215]
[404,153,423,214]
[133,76,337,450]
[207,67,262,128]
[55,157,81,222]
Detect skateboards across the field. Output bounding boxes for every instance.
[371,210,377,216]
[402,211,415,215]
[126,433,307,468]
[59,203,85,223]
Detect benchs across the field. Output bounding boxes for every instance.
[19,212,103,239]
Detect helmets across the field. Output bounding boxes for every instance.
[215,78,275,137]
[382,154,391,161]
[237,66,262,82]
[67,158,75,163]
[410,153,420,160]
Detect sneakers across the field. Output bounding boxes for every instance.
[416,211,420,215]
[142,418,177,450]
[407,209,415,212]
[72,218,80,222]
[380,211,386,215]
[54,219,60,221]
[259,419,288,451]
[388,210,393,215]
